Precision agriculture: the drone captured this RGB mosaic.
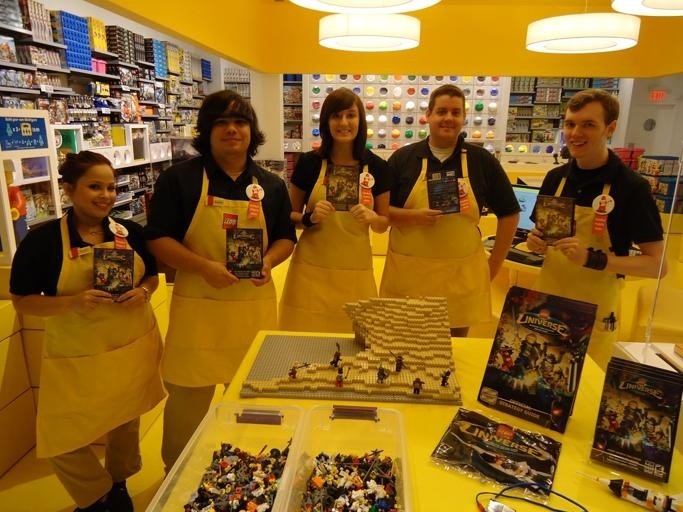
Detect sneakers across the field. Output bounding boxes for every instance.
[72,499,106,512]
[106,479,131,512]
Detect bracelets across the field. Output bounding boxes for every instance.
[583,247,608,271]
[139,286,152,305]
[302,212,315,228]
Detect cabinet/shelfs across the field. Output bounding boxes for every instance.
[0,19,214,225]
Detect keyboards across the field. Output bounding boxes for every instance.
[490,245,544,266]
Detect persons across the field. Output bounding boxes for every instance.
[278,87,390,332]
[377,367,385,383]
[527,87,668,368]
[335,368,344,388]
[379,84,522,337]
[144,90,297,480]
[441,371,450,386]
[413,378,422,394]
[395,356,403,372]
[9,151,164,512]
[330,351,341,367]
[288,367,297,378]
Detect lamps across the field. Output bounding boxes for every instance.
[288,0,443,53]
[524,0,683,55]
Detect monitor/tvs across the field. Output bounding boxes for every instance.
[508,183,541,239]
